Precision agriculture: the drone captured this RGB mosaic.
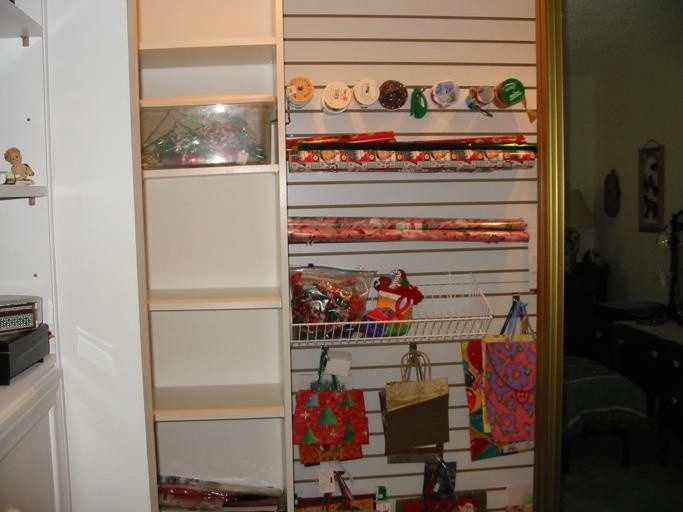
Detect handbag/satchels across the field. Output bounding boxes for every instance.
[376,348,453,466]
[292,352,370,469]
[459,294,538,463]
[292,472,379,512]
[395,462,490,512]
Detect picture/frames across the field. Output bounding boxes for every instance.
[637,145,666,233]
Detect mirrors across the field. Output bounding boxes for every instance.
[666,209,683,326]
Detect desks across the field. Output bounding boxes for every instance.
[564,260,610,333]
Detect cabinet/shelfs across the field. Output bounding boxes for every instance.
[125,0,296,511]
[615,317,683,467]
[0,0,73,511]
[284,0,565,511]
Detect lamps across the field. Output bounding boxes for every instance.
[565,191,597,264]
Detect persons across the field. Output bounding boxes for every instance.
[4,147,34,180]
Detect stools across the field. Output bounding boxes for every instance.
[563,347,649,486]
